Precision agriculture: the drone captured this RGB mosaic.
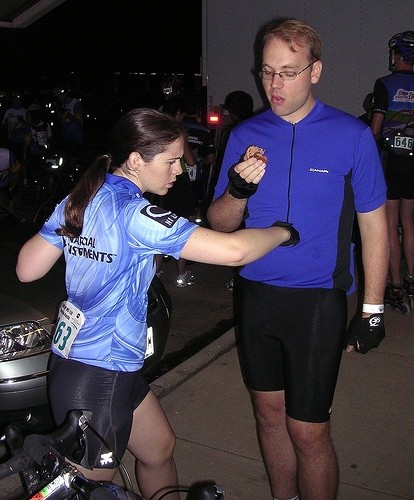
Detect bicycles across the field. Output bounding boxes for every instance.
[0,410,227,500]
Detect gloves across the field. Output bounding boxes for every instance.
[272,220,301,247]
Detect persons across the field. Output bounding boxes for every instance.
[224,90,255,293]
[370,30,414,314]
[206,18,390,500]
[16,107,299,500]
[52,87,83,129]
[2,91,28,137]
[155,98,196,288]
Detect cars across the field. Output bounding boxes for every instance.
[0,201,172,411]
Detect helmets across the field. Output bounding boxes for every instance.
[220,91,254,113]
[389,31,414,61]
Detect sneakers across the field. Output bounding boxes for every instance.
[154,271,164,279]
[175,271,196,288]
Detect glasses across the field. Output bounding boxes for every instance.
[260,60,318,81]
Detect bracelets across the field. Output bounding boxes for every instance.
[362,304,385,314]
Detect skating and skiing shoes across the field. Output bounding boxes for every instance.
[384,284,410,315]
[402,275,414,310]
